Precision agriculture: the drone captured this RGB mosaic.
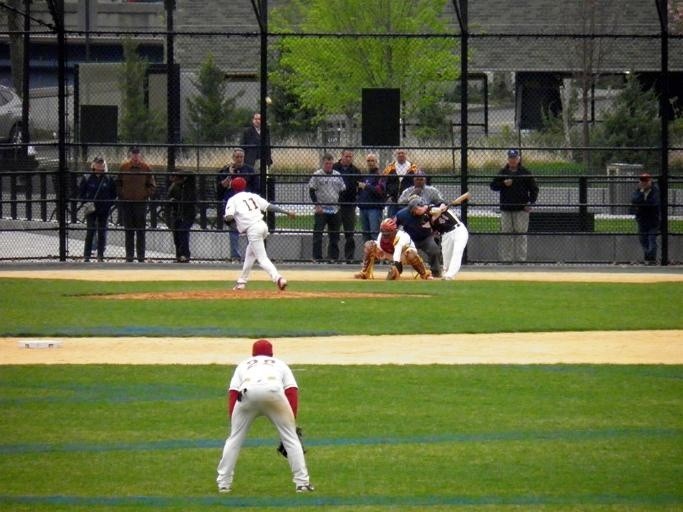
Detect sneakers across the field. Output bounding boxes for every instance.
[355,272,369,279]
[233,285,244,290]
[422,274,432,279]
[277,278,287,289]
[295,484,313,492]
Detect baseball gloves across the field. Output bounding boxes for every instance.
[278,427,306,458]
[388,266,400,281]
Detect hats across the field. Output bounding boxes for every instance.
[508,148,518,157]
[231,176,245,191]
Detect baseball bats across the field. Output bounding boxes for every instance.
[442,192,469,211]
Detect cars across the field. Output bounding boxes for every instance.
[1,85,33,144]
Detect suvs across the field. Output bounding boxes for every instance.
[316,104,403,146]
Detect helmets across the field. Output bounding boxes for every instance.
[380,171,427,236]
[252,340,272,355]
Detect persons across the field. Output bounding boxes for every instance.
[490,148,539,264]
[116,148,157,262]
[164,172,196,263]
[217,340,314,493]
[216,148,260,264]
[222,176,296,290]
[240,113,273,174]
[80,156,117,262]
[307,148,469,281]
[629,174,660,266]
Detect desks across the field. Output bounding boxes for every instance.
[49,174,217,229]
